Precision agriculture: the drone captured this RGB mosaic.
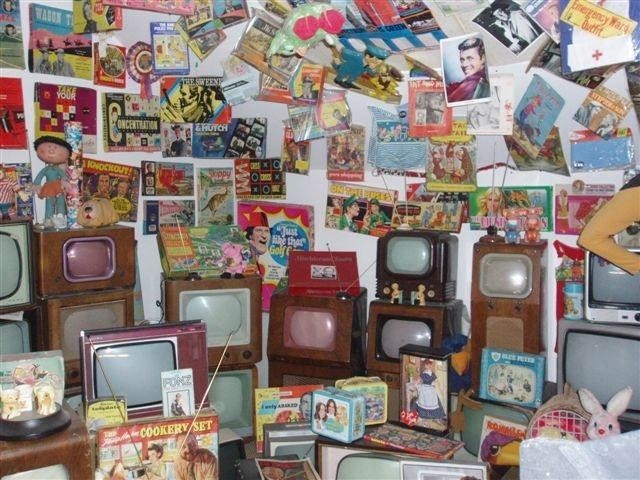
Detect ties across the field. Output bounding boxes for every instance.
[187,463,195,480]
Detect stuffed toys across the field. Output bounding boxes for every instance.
[78,197,124,227]
[213,242,252,279]
[577,387,635,441]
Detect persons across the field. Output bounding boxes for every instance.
[369,199,390,227]
[383,284,429,308]
[170,393,188,417]
[184,128,192,137]
[328,145,341,170]
[146,164,155,177]
[3,0,15,12]
[108,180,132,203]
[341,200,371,234]
[247,225,271,254]
[185,138,191,147]
[445,38,490,104]
[84,171,114,200]
[34,48,56,75]
[487,3,539,53]
[405,357,448,426]
[34,134,73,231]
[138,442,167,480]
[473,188,510,225]
[4,23,16,38]
[221,0,242,14]
[323,399,337,432]
[295,74,319,101]
[147,178,153,186]
[312,402,326,432]
[176,431,215,480]
[300,391,316,416]
[165,126,188,159]
[334,152,354,171]
[51,48,77,78]
[195,83,233,128]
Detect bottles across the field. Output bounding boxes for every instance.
[562,280,584,319]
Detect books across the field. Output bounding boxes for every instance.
[252,382,328,454]
[363,422,466,460]
[160,367,196,419]
